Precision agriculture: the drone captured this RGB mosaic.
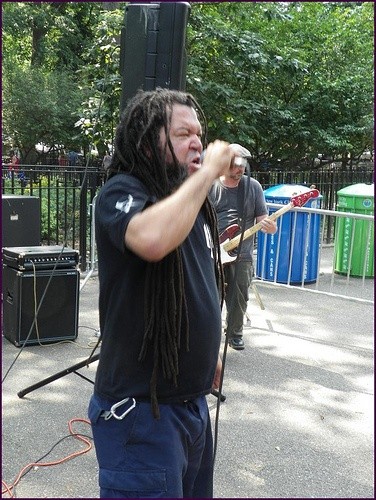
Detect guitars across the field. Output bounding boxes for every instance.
[217,186,322,269]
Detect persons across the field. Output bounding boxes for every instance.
[4,149,26,184]
[87,85,239,500]
[207,153,278,350]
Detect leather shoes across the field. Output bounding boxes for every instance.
[228,335,246,349]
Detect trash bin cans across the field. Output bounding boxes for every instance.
[255,183,323,283]
[334,183,374,278]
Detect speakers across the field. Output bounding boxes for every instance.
[119,2,190,118]
[2,194,40,248]
[3,265,80,348]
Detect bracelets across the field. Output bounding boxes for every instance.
[271,225,278,235]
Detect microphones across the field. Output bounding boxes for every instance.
[201,150,245,168]
